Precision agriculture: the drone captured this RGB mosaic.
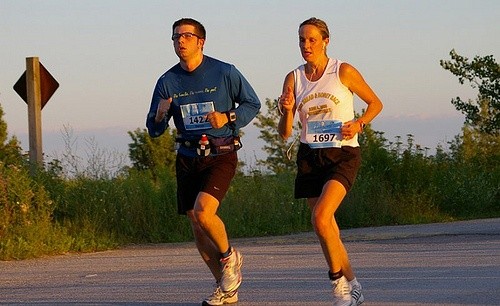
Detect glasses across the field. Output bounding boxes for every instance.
[172,32,202,41]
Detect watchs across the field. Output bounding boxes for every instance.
[226,110,237,124]
[357,119,365,131]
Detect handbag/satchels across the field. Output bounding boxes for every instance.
[178,135,242,155]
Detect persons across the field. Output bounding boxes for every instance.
[276,17,383,306]
[146,19,261,305]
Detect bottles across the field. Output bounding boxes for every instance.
[197,135,210,156]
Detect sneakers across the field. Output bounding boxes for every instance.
[218,245,244,294]
[350,282,365,306]
[202,281,239,306]
[330,275,352,306]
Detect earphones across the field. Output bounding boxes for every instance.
[323,42,325,49]
